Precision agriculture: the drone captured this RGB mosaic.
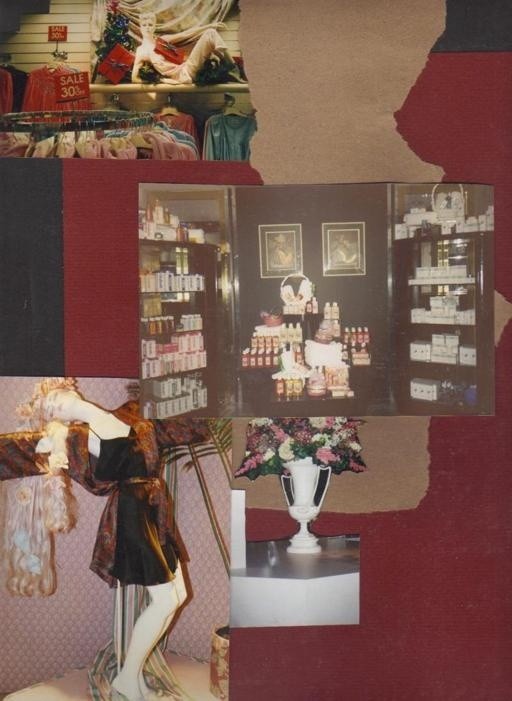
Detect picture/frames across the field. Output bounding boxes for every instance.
[258,223,304,279]
[321,221,367,277]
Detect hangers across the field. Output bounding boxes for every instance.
[223,96,245,117]
[0,110,157,143]
[45,53,79,73]
[156,94,180,116]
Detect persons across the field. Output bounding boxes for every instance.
[134,12,235,85]
[1,377,188,700]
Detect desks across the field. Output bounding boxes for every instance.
[230,534,360,629]
[238,313,371,417]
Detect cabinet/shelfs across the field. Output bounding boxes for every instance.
[137,238,218,419]
[392,232,495,415]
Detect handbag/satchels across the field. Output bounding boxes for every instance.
[197,60,228,85]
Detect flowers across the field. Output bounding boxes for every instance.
[233,417,368,480]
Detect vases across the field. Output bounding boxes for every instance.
[279,457,332,553]
